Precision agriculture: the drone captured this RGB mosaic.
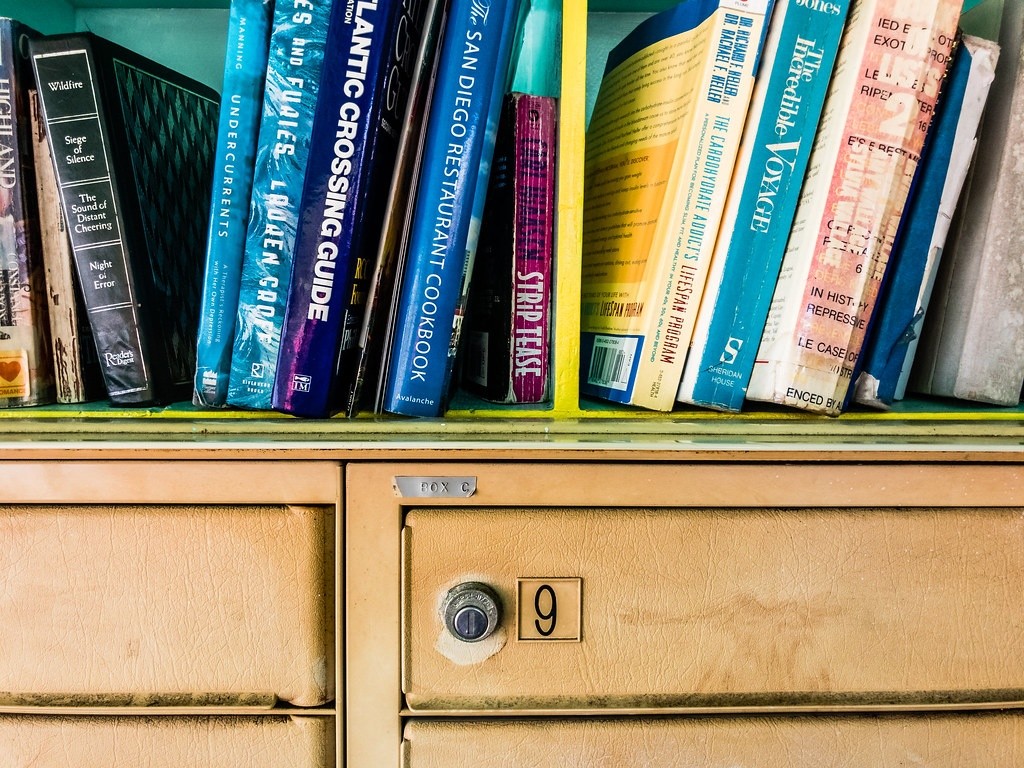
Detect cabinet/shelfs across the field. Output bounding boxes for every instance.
[0,442,1024,768]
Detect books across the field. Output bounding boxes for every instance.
[0,0,1024,416]
